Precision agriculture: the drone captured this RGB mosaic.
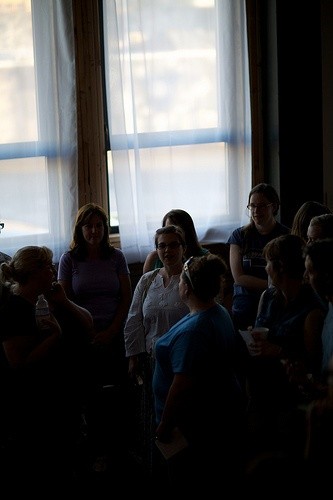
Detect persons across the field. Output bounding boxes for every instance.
[123,183,333,478]
[62,203,133,474]
[0,246,78,375]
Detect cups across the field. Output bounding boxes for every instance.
[249,328,270,342]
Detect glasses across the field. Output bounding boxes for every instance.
[157,242,183,249]
[247,203,274,210]
[183,255,194,289]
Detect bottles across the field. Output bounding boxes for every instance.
[34,294,50,329]
